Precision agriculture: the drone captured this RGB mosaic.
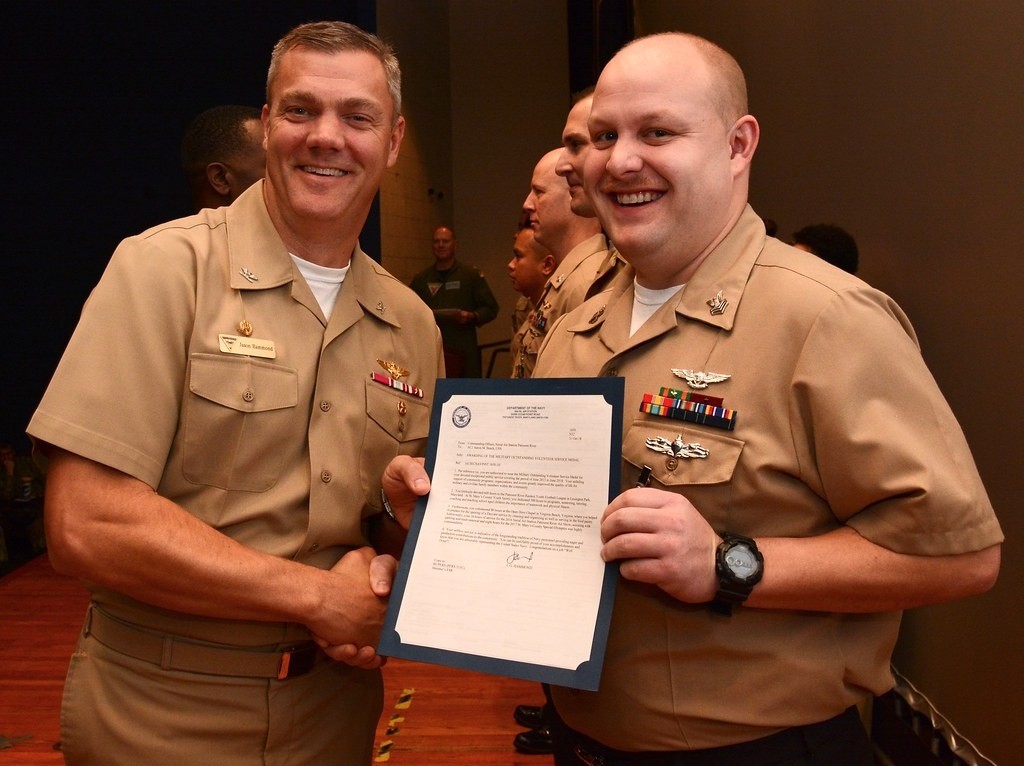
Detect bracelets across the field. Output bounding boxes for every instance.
[381,489,395,520]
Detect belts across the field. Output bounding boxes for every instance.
[87,602,318,680]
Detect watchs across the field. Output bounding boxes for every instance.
[711,531,765,614]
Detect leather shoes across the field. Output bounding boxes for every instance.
[513,704,553,754]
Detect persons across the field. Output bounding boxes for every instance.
[556,88,627,300]
[324,30,1004,766]
[409,227,499,379]
[792,224,859,274]
[513,144,609,379]
[507,211,557,358]
[24,20,446,766]
[184,106,267,214]
